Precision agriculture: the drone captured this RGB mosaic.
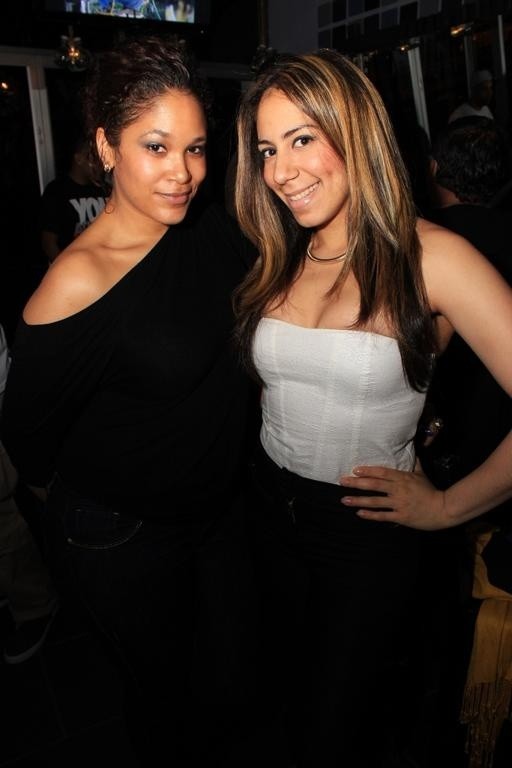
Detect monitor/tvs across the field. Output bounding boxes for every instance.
[46,0,211,32]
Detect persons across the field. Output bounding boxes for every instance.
[0,37,512,768]
